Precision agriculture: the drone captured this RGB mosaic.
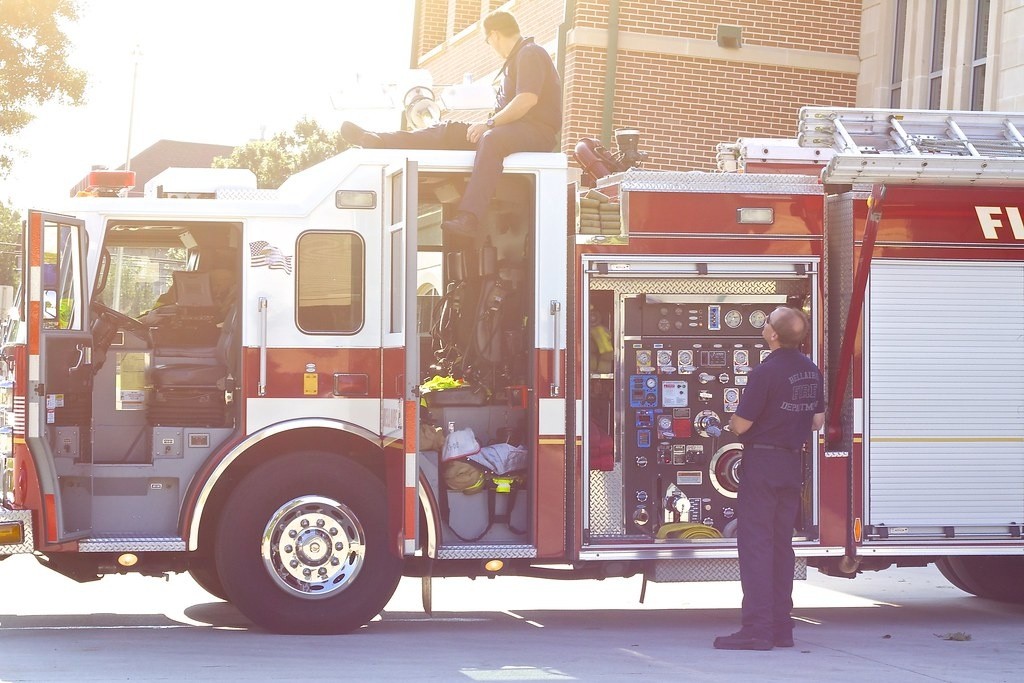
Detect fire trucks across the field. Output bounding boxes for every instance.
[1,105,1024,637]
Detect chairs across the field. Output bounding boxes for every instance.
[150,295,240,386]
[149,294,235,378]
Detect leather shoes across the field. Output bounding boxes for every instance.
[341,121,386,149]
[440,212,482,240]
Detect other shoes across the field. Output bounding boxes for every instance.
[712,622,794,651]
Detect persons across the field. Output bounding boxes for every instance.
[713,307,825,651]
[341,12,561,238]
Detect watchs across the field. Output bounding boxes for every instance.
[486,118,495,129]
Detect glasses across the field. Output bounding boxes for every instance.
[766,308,777,333]
[484,32,493,44]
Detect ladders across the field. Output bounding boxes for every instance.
[713,107,1024,185]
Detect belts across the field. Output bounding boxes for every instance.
[744,442,802,454]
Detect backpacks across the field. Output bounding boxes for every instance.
[444,442,531,494]
